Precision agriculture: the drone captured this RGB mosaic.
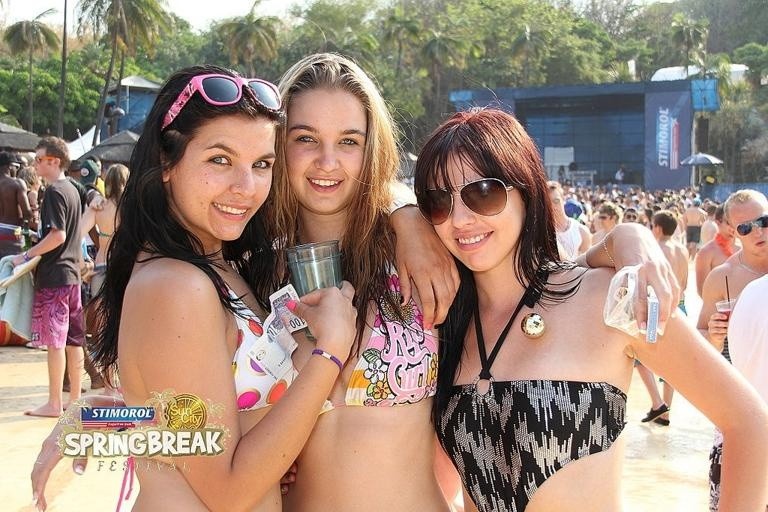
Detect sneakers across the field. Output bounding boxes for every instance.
[642,403,670,422]
[647,412,670,425]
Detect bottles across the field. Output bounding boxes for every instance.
[19,213,31,251]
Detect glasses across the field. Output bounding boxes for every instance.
[737,215,768,236]
[35,156,55,165]
[162,72,282,130]
[627,215,637,219]
[417,178,515,225]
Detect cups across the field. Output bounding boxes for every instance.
[715,299,738,320]
[287,240,343,338]
